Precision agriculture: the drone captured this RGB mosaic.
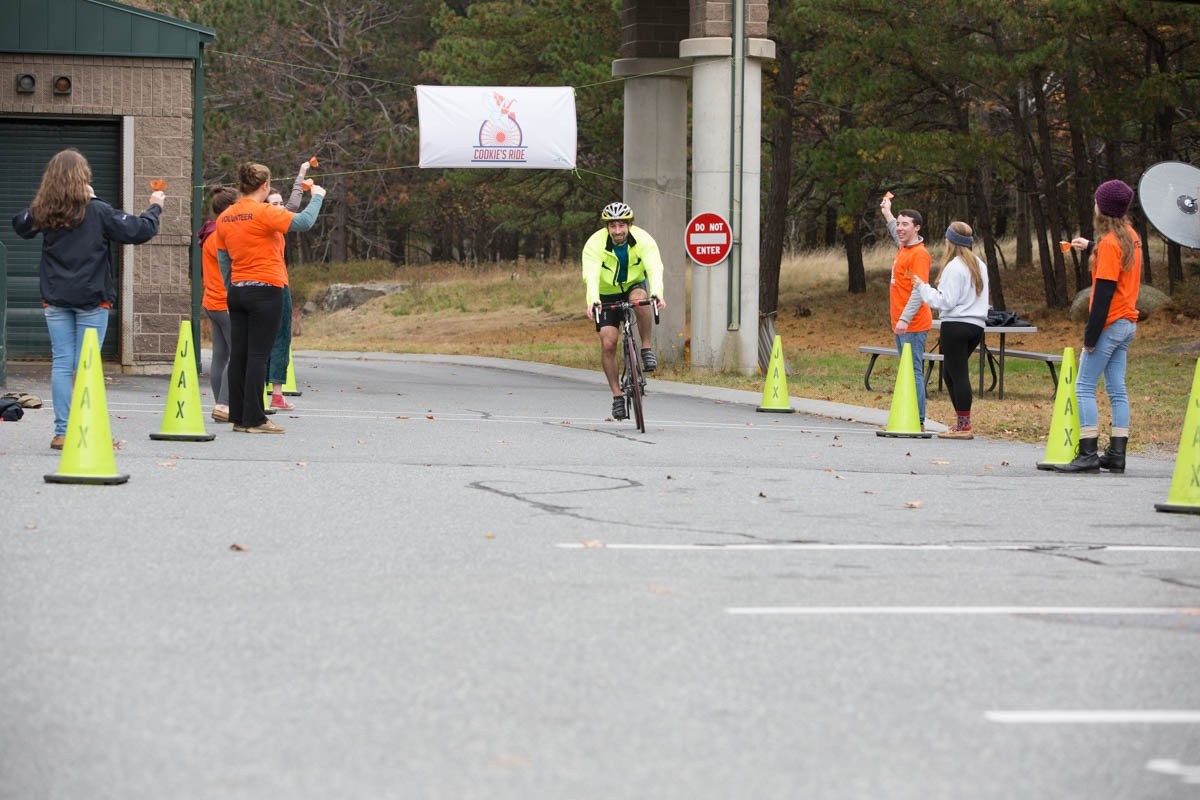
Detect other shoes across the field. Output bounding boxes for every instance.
[51,437,64,449]
[232,423,247,432]
[881,425,925,432]
[938,429,973,439]
[247,420,286,433]
[211,404,229,422]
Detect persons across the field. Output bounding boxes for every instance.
[216,161,325,434]
[582,202,665,420]
[264,162,310,410]
[1053,180,1141,474]
[195,183,242,422]
[880,197,933,431]
[11,151,165,449]
[913,222,989,439]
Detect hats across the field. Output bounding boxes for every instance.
[1095,180,1134,217]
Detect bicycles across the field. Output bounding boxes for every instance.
[592,292,660,435]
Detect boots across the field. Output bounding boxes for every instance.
[1099,436,1128,473]
[1054,438,1100,473]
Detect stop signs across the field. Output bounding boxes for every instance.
[683,213,733,267]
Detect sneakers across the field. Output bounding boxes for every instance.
[270,394,295,410]
[641,351,657,368]
[612,398,626,418]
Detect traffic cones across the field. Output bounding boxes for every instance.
[42,328,131,484]
[1154,358,1199,513]
[756,334,797,413]
[267,338,301,396]
[263,381,279,415]
[147,319,220,443]
[878,343,936,439]
[1036,345,1085,471]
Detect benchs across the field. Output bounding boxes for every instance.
[973,347,1063,399]
[857,346,944,400]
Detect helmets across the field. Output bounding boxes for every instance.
[600,202,634,220]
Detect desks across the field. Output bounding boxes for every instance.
[931,320,1037,399]
